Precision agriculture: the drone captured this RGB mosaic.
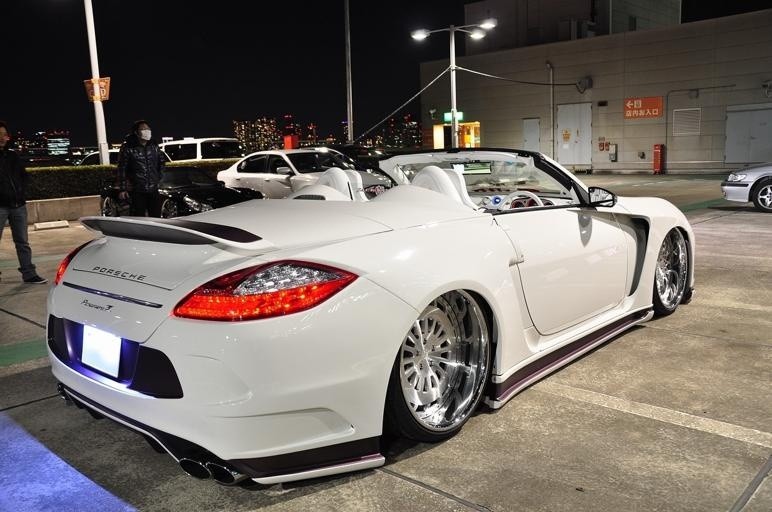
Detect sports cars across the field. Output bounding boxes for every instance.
[42,147,698,490]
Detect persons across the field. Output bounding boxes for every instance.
[0,123,49,283]
[118,120,166,218]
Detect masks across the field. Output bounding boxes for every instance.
[141,130,152,140]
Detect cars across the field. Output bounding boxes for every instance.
[720,164,772,214]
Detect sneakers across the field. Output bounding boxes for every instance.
[23,275,48,284]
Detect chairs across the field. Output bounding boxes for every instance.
[344,169,368,203]
[412,166,461,203]
[316,167,353,198]
[445,169,479,210]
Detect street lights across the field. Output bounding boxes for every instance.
[412,18,500,149]
